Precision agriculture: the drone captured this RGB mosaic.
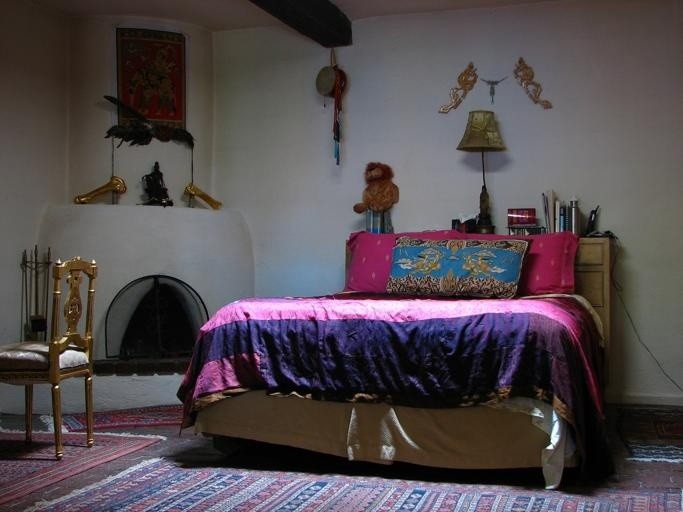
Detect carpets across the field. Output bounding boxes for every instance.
[0,404,682,511]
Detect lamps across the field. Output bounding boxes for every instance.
[455,110,507,234]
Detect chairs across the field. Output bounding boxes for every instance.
[0,254,99,461]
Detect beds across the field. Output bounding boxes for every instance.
[186,234,610,486]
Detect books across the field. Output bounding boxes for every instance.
[540,186,571,236]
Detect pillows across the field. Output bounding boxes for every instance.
[342,229,581,300]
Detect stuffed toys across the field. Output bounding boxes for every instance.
[349,159,400,215]
